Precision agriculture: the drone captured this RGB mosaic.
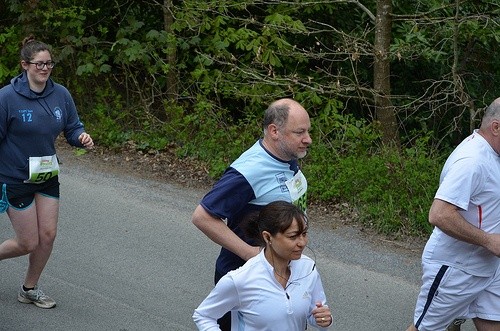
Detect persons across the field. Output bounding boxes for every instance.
[192,99,333,331]
[0,35,94,309]
[405,97,500,331]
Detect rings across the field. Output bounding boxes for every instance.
[321,317,326,322]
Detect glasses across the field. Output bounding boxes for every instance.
[26,61,56,70]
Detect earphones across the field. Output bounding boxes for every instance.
[268,237,270,241]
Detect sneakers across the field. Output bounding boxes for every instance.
[17,284,56,309]
